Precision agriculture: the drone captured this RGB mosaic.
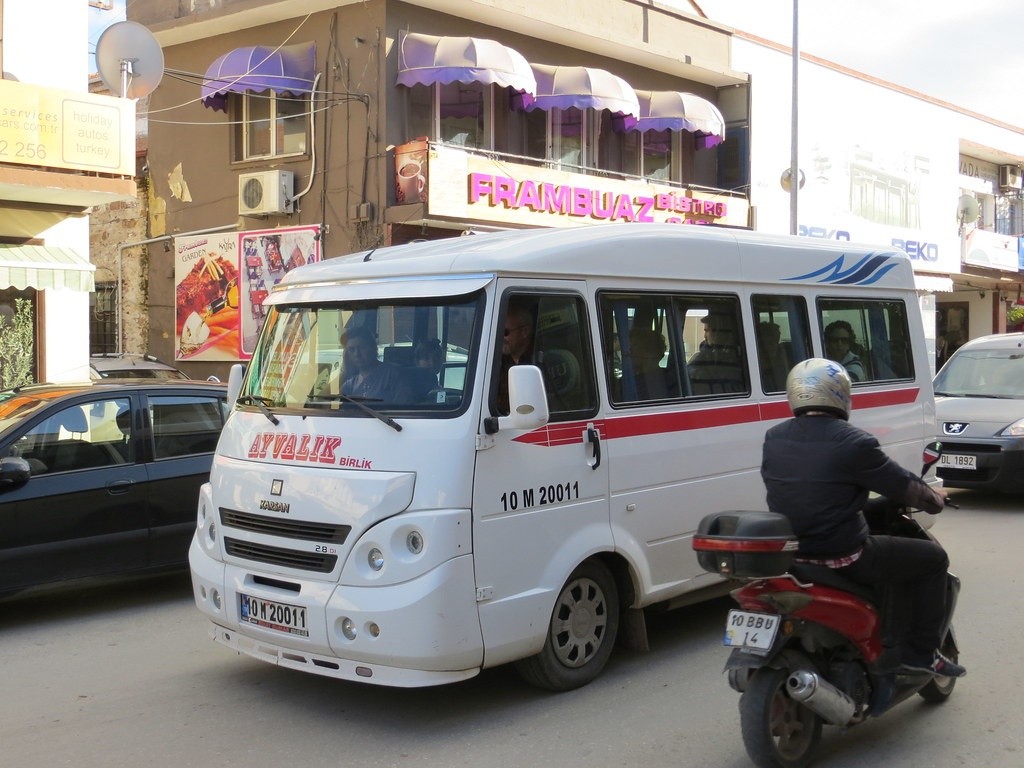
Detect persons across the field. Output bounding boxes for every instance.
[613,329,670,402]
[338,327,412,402]
[760,358,967,678]
[497,303,532,415]
[825,320,866,382]
[686,314,787,396]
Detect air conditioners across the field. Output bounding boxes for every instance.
[238,170,293,217]
[1000,164,1022,191]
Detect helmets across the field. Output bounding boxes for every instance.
[786,358,852,420]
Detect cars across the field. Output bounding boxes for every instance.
[926,330,1024,500]
[88,350,190,382]
[0,378,232,601]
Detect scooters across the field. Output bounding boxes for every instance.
[689,434,964,767]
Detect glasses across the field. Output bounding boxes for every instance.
[829,337,850,346]
[504,323,527,337]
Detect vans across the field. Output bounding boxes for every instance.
[187,219,944,694]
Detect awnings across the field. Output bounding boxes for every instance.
[201,39,317,114]
[525,63,641,133]
[0,244,95,292]
[394,29,537,111]
[633,89,725,151]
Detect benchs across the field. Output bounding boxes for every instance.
[158,411,220,457]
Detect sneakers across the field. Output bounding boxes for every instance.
[901,647,967,678]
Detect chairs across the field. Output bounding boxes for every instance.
[103,406,132,464]
[539,351,587,408]
[42,406,94,474]
